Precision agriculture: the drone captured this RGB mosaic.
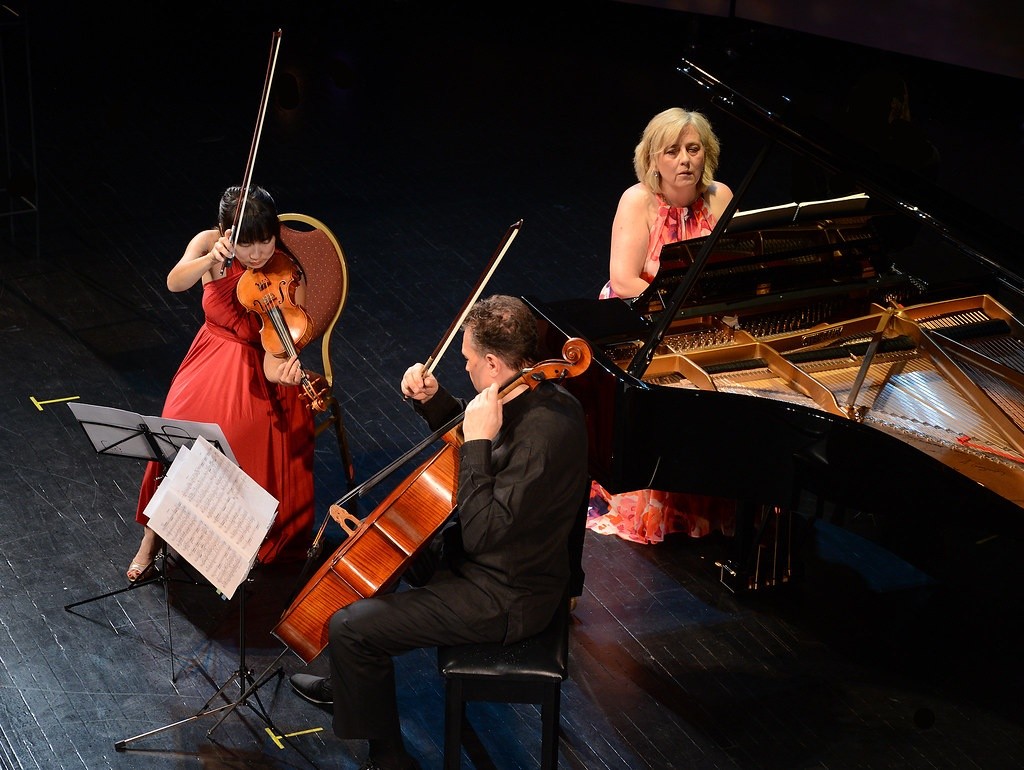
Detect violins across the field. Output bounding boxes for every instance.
[235,252,329,415]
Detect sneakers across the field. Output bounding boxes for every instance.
[288,672,333,710]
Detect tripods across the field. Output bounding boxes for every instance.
[65,419,323,770]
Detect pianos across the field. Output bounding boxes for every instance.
[529,184,1024,599]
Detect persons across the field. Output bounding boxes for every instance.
[582,107,740,544]
[126,184,321,583]
[289,294,589,770]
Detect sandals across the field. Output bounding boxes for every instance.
[126,527,163,582]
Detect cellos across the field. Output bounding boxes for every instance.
[206,335,594,735]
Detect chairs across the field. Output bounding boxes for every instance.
[278,214,354,487]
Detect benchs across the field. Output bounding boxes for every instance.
[436,474,593,770]
